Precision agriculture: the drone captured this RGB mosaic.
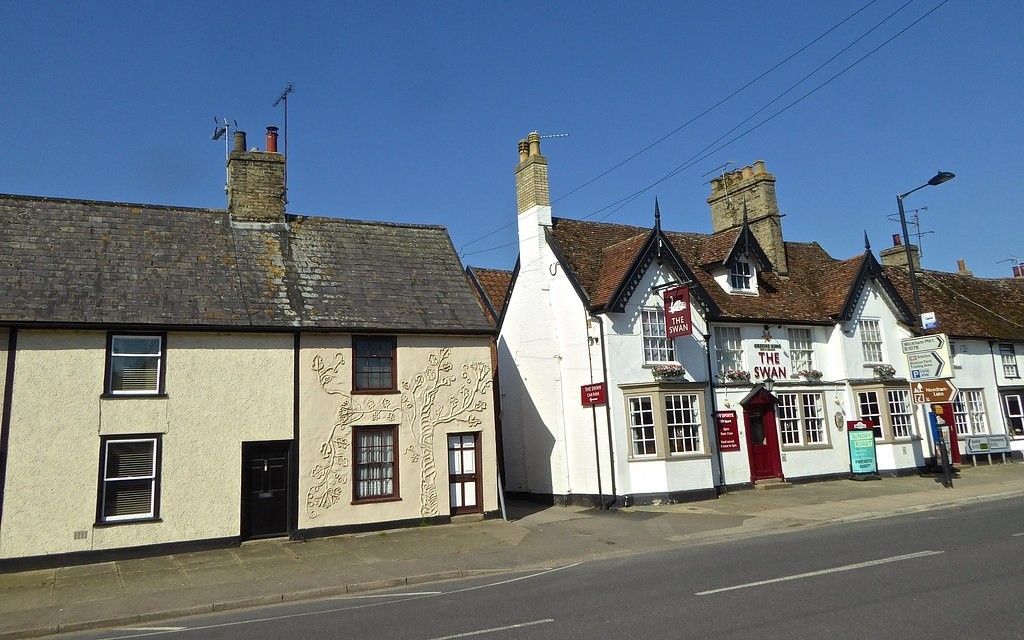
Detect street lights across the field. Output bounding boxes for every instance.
[896,170,956,489]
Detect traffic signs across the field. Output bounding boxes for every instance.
[900,332,955,381]
[909,379,960,403]
